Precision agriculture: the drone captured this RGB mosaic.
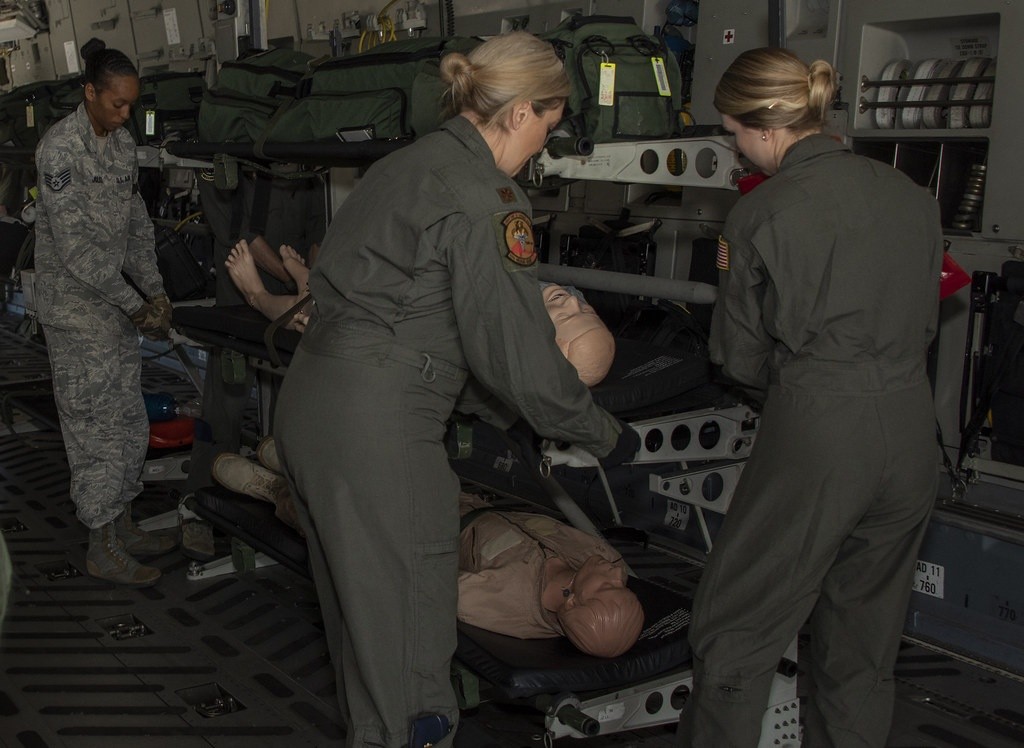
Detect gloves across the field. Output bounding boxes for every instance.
[507,418,544,463]
[598,419,641,471]
[150,293,171,317]
[130,303,171,342]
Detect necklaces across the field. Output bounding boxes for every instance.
[561,573,575,598]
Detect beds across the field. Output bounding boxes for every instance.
[182,484,799,741]
[160,290,761,469]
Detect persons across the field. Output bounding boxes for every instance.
[179,167,332,563]
[212,434,646,658]
[270,29,642,748]
[224,238,616,387]
[34,38,177,584]
[675,47,944,748]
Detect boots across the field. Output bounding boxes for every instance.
[116,515,179,556]
[85,521,161,587]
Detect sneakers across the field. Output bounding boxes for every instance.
[182,522,216,562]
[257,436,281,472]
[213,453,289,504]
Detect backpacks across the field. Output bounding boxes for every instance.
[0,15,686,148]
